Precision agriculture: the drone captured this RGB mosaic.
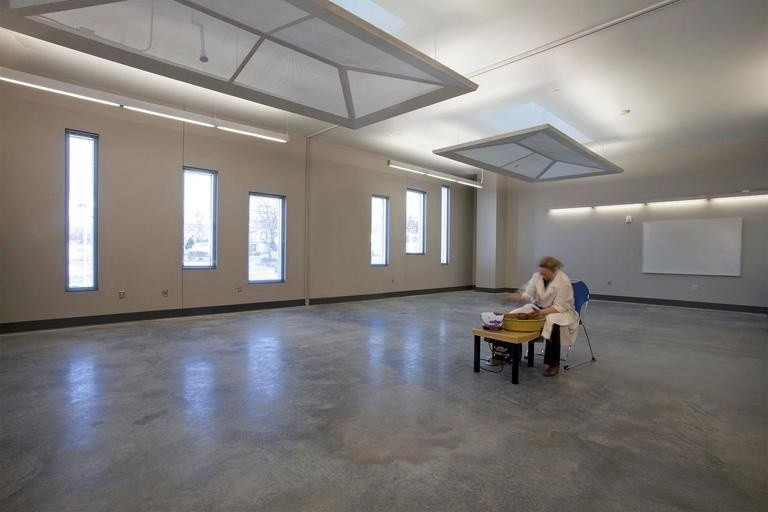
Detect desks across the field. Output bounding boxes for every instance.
[471,327,541,384]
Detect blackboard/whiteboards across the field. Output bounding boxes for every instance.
[642,217,743,276]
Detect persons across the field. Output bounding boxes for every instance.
[487,255,580,377]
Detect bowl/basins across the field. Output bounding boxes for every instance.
[504,313,546,332]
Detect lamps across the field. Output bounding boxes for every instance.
[387,127,485,189]
[0,65,290,144]
[191,8,209,63]
[546,190,768,214]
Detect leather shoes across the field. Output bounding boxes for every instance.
[543,365,560,377]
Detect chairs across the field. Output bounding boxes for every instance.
[523,278,595,370]
